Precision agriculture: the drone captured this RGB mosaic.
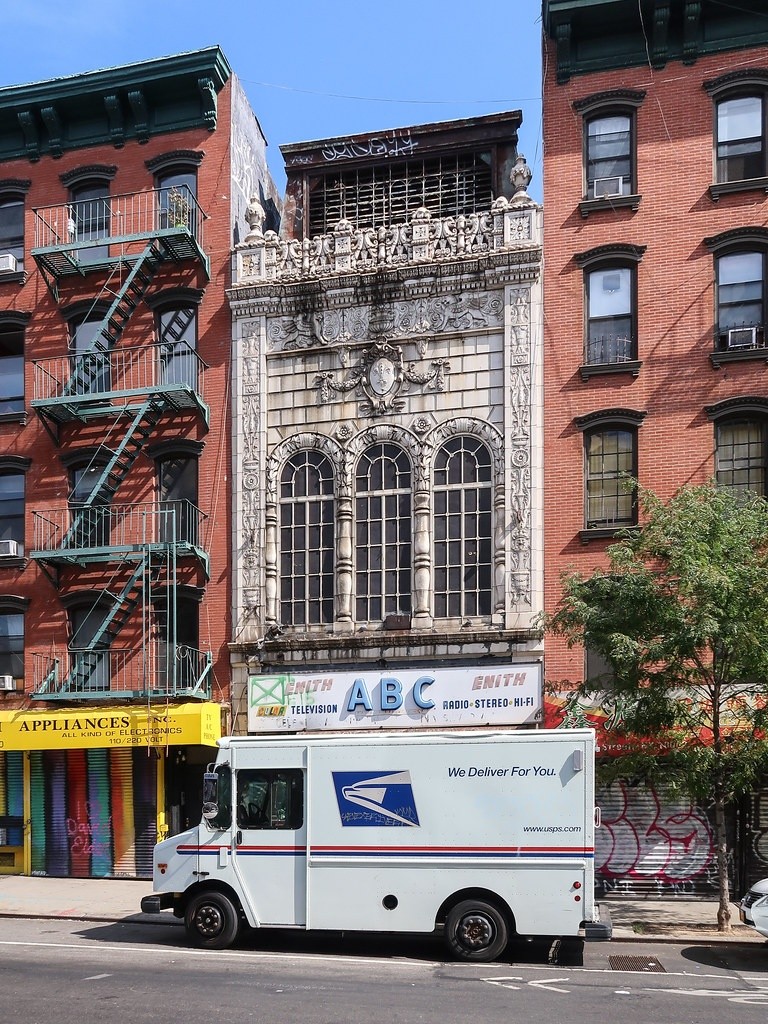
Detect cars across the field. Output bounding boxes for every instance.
[738,878,768,937]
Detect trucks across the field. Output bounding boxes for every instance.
[139,727,614,962]
